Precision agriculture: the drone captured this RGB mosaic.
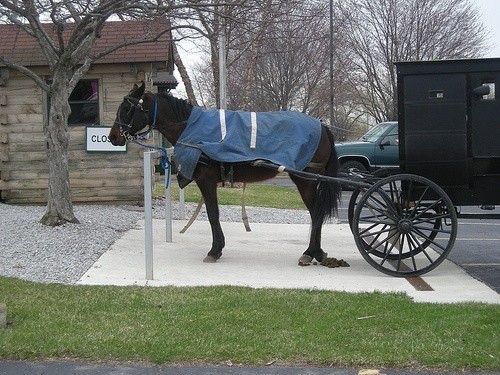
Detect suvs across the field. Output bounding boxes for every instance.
[333,120,398,190]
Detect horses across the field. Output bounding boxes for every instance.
[108,81,342,266]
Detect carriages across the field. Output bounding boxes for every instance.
[109,57,500,277]
